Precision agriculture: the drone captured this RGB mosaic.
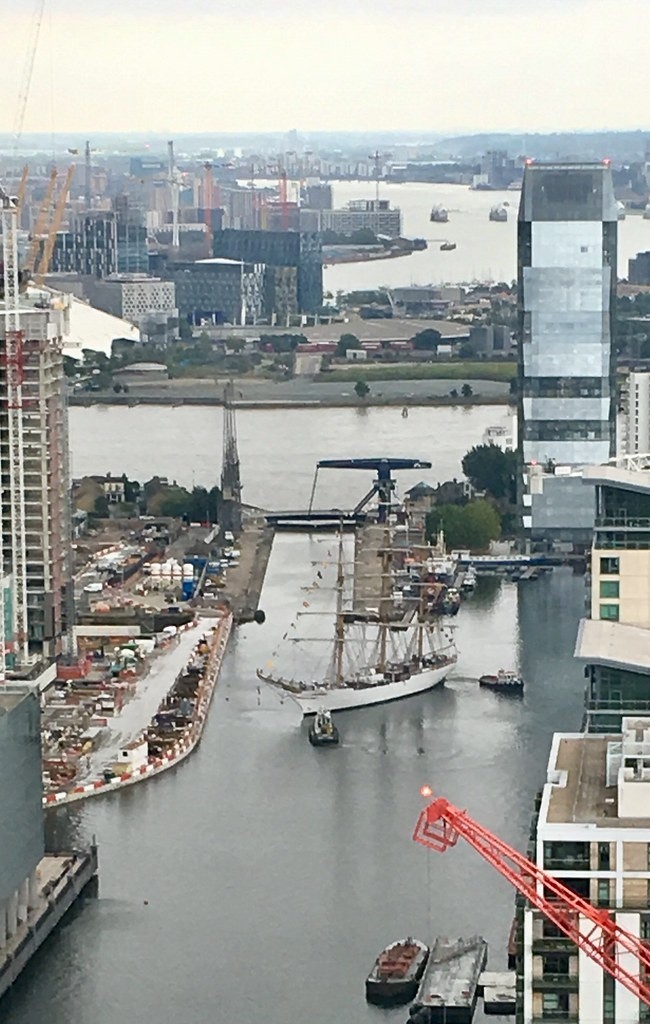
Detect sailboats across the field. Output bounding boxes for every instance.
[254,476,461,717]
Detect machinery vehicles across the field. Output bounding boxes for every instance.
[412,787,650,1006]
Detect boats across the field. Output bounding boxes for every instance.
[477,667,525,694]
[363,935,430,998]
[306,704,340,747]
